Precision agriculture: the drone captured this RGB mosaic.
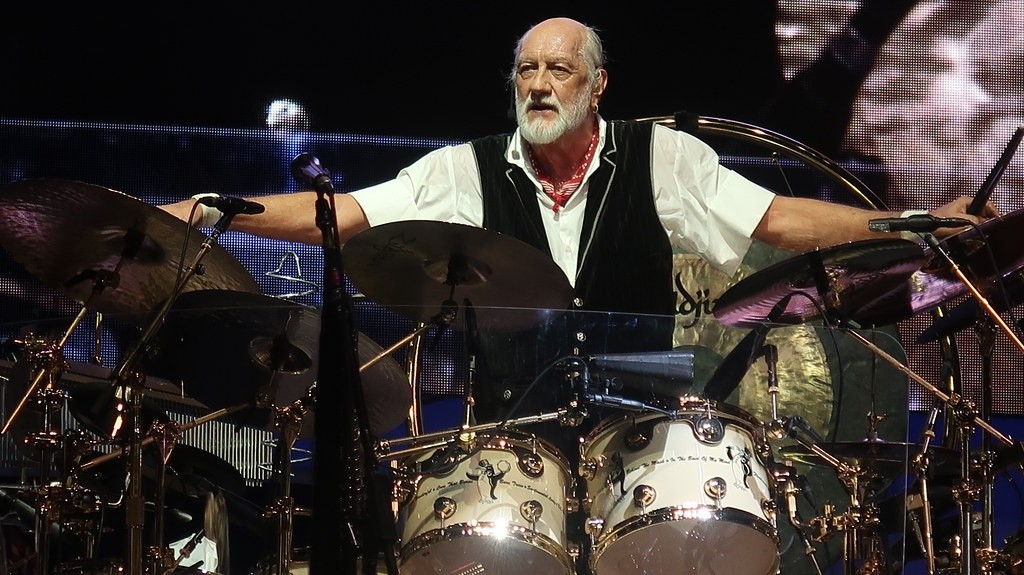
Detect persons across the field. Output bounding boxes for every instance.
[158,18,1004,466]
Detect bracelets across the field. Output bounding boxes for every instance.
[900,210,929,243]
[191,193,222,229]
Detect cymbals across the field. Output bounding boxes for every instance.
[711,235,926,336]
[780,435,953,472]
[826,209,1024,331]
[0,175,260,314]
[339,219,567,329]
[914,269,1024,346]
[171,293,411,446]
[5,370,249,541]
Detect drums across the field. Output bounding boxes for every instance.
[575,400,783,575]
[390,424,575,574]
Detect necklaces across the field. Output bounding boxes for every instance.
[525,123,599,214]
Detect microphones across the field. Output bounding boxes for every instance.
[465,301,485,379]
[703,294,792,403]
[578,367,590,411]
[868,214,973,233]
[798,475,821,517]
[290,152,335,195]
[198,197,265,215]
